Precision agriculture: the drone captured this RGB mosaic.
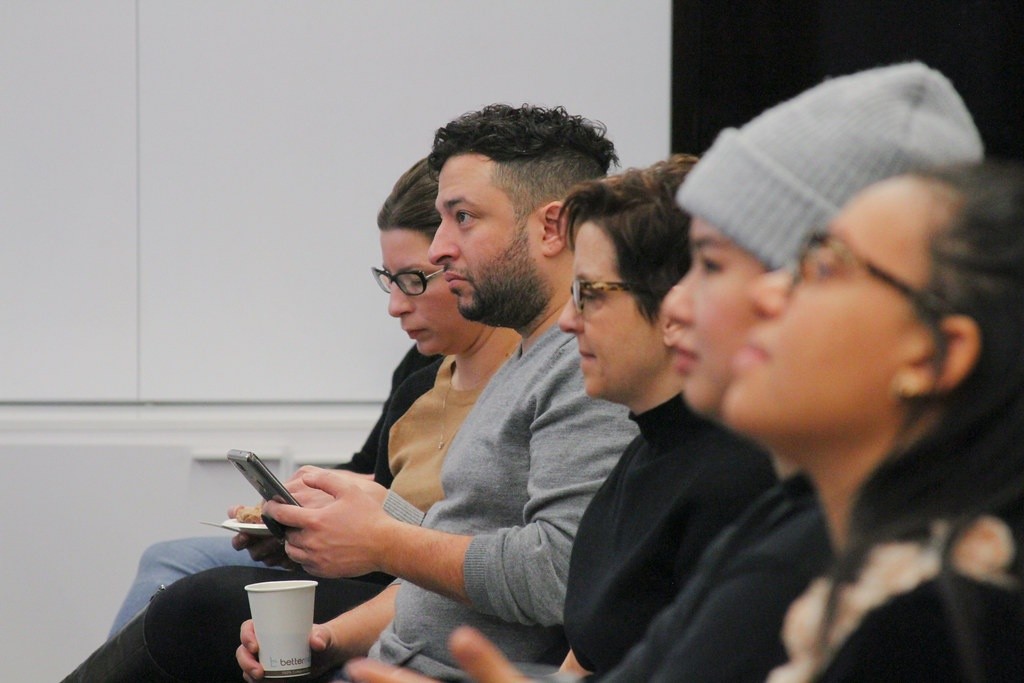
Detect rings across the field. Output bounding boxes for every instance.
[297,560,305,566]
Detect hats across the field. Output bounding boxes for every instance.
[676,62,983,283]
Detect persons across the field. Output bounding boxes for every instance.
[234,104,642,683]
[543,152,779,683]
[61,152,522,683]
[595,58,1024,683]
[329,156,1024,683]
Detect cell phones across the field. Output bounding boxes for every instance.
[226,449,304,531]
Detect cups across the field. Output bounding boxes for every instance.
[244,580,318,679]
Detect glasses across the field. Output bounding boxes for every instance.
[569,280,634,314]
[793,232,939,319]
[371,265,446,296]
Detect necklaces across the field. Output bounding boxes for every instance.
[439,355,489,447]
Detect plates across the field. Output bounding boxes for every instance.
[221,519,273,536]
